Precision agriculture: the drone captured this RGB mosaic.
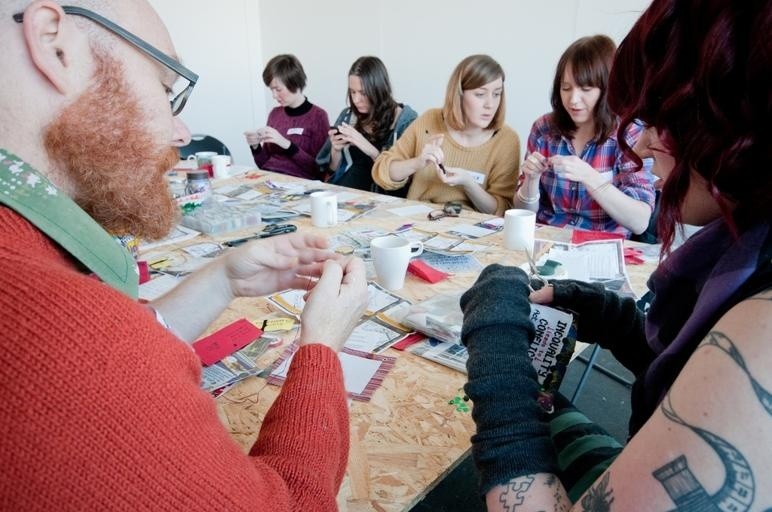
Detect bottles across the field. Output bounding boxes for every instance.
[183,172,213,194]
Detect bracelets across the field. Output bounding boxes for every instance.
[516,186,542,204]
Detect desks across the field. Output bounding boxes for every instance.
[137,164,680,512]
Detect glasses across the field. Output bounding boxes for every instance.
[14,7,198,115]
[428,203,461,221]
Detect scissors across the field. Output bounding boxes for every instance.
[524,247,552,292]
[226,224,297,245]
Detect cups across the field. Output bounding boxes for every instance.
[500,208,539,253]
[210,155,231,181]
[370,235,426,289]
[188,151,217,177]
[308,189,340,228]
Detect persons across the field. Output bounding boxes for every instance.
[315,57,418,198]
[372,54,519,217]
[243,54,329,180]
[460,0,772,512]
[0,0,369,512]
[513,34,656,240]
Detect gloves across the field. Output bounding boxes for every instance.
[459,263,559,502]
[531,276,645,379]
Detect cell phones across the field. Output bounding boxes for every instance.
[330,126,342,141]
